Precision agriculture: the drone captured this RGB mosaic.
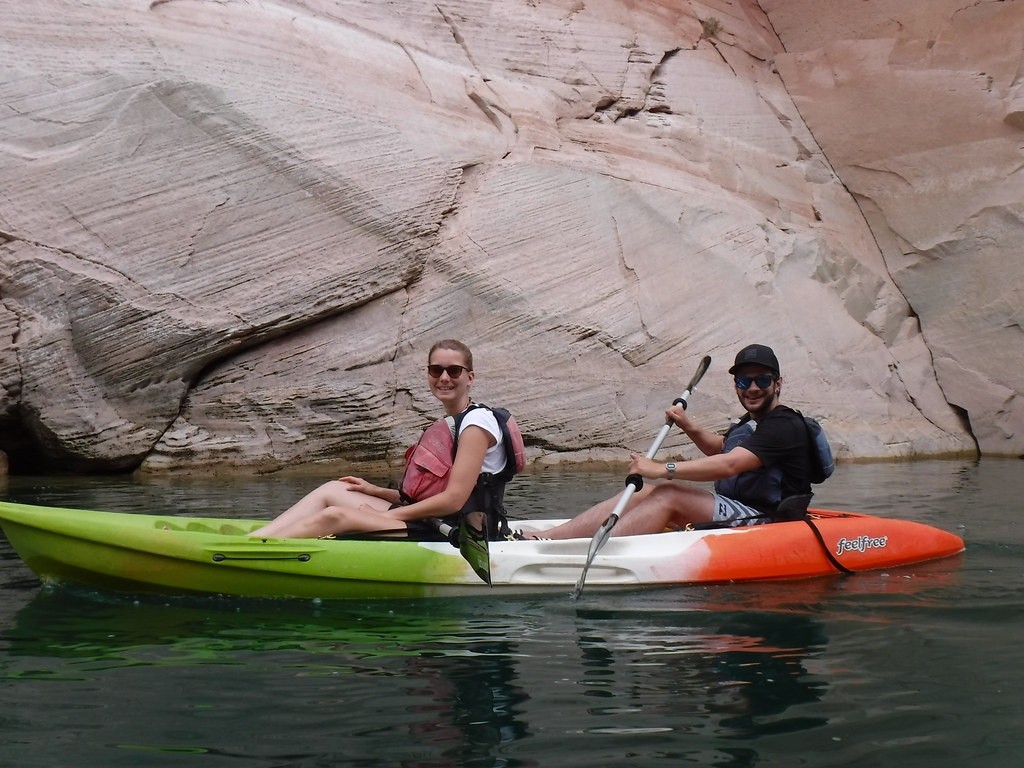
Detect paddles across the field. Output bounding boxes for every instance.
[574,356,713,594]
[388,478,493,589]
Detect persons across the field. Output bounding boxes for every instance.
[163,338,510,539]
[497,344,813,542]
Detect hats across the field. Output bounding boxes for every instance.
[728,344,780,380]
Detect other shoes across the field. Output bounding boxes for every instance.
[497,519,552,541]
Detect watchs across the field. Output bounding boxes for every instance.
[665,462,675,480]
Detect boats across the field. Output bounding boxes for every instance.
[1,497,971,603]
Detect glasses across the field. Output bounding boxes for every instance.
[734,375,777,389]
[427,365,470,378]
